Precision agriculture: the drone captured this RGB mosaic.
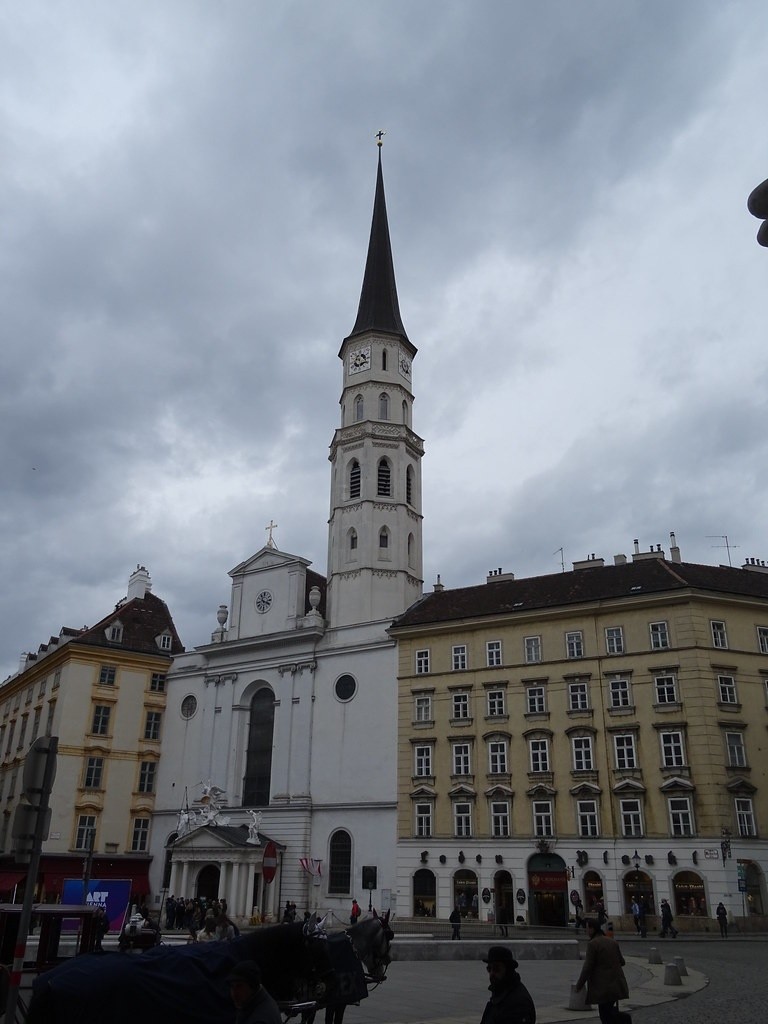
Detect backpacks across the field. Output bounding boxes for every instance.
[357,906,361,916]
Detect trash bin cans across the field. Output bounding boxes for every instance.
[487,910,494,924]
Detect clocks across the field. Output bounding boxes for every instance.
[255,590,274,614]
[349,346,371,375]
[399,351,412,383]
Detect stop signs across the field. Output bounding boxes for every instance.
[263,842,278,884]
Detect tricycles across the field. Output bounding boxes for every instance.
[119,928,161,955]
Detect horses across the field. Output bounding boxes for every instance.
[23,907,394,1024]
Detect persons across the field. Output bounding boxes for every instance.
[199,780,221,825]
[479,946,536,1024]
[575,920,632,1024]
[658,898,678,938]
[222,960,282,1023]
[166,895,240,943]
[631,898,646,937]
[128,894,149,920]
[717,902,727,938]
[286,900,297,919]
[494,886,509,937]
[575,900,584,928]
[176,810,188,837]
[246,810,259,837]
[449,888,478,940]
[680,896,707,916]
[28,892,61,935]
[94,907,110,953]
[414,897,436,917]
[351,899,361,925]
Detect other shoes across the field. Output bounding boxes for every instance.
[672,931,678,938]
[721,935,724,937]
[659,932,665,938]
[633,931,641,935]
[726,935,728,937]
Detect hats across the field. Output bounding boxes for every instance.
[661,898,666,901]
[352,898,358,904]
[718,902,724,906]
[482,947,518,970]
